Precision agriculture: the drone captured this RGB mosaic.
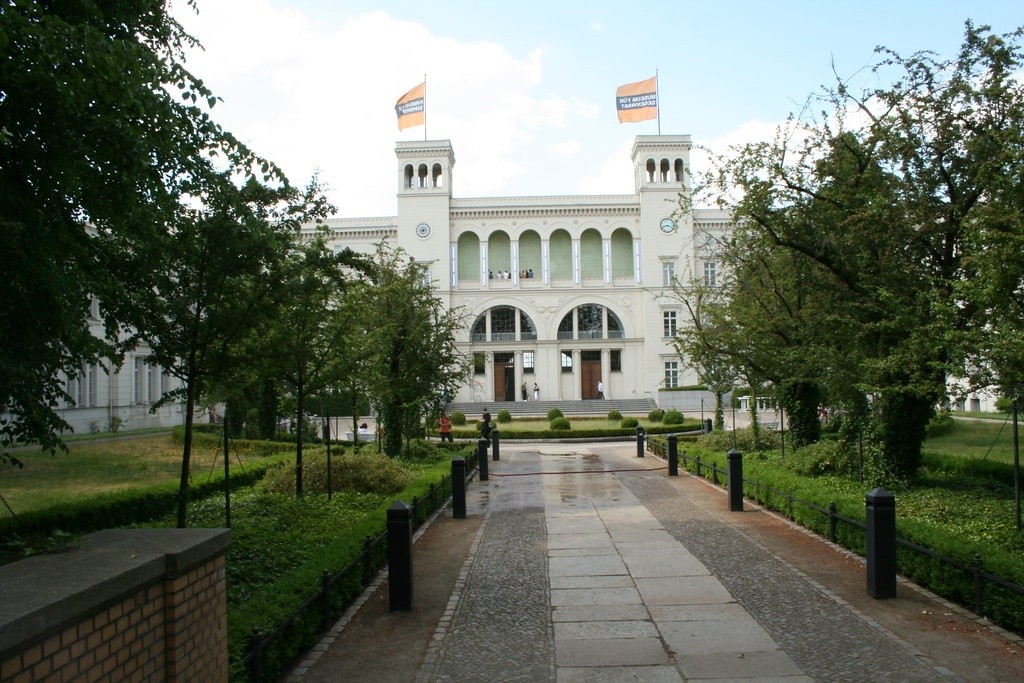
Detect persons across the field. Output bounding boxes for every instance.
[439,413,454,443]
[487,268,535,280]
[521,381,527,401]
[533,383,539,401]
[477,408,492,447]
[595,379,605,400]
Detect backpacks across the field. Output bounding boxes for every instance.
[438,417,444,427]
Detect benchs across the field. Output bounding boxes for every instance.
[757,421,779,430]
[345,432,376,442]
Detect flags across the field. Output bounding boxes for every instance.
[616,73,660,124]
[395,80,426,130]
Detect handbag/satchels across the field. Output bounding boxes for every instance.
[488,422,496,428]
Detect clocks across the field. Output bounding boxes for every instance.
[660,218,674,233]
